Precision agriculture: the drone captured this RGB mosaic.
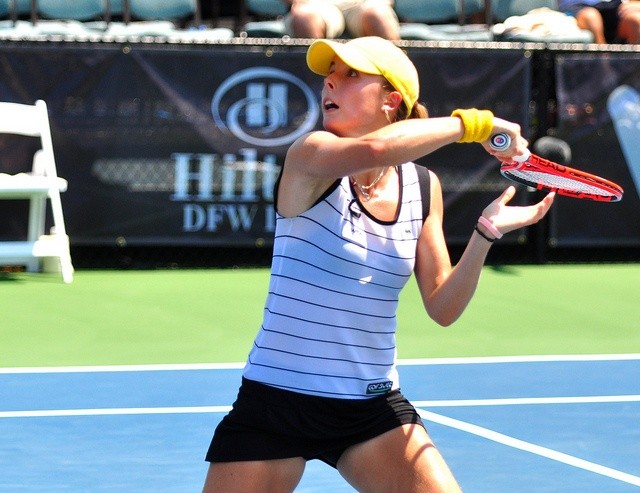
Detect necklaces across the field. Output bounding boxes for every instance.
[348,167,386,201]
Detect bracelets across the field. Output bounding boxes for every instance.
[473,225,495,245]
[478,215,503,240]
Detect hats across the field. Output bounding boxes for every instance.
[307,35,419,120]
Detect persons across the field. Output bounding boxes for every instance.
[203,35,555,493]
[288,0,400,45]
[556,0,640,45]
